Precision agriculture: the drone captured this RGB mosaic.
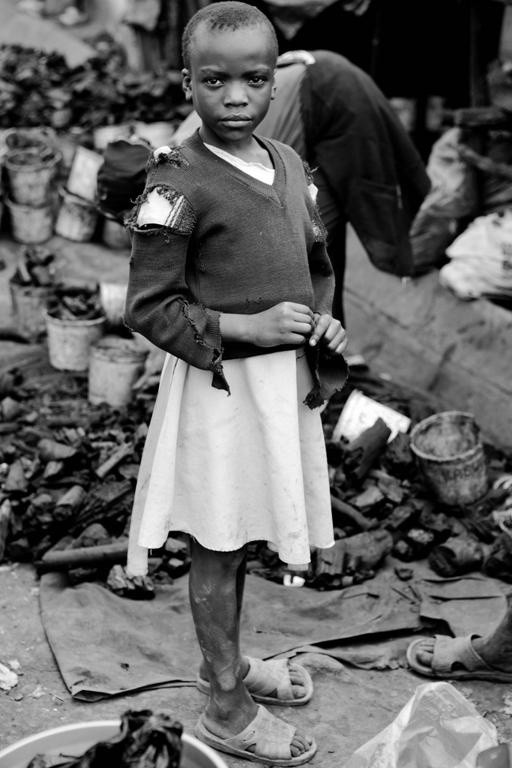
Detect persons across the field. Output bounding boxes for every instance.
[406,594,512,683]
[122,1,348,768]
[165,2,430,423]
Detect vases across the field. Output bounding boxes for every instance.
[0,121,177,415]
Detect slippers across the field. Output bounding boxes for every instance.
[407,633,512,682]
[194,703,318,767]
[196,658,313,707]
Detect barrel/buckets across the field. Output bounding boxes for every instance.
[408,410,487,506]
[329,388,411,454]
[0,94,445,412]
[0,718,228,768]
